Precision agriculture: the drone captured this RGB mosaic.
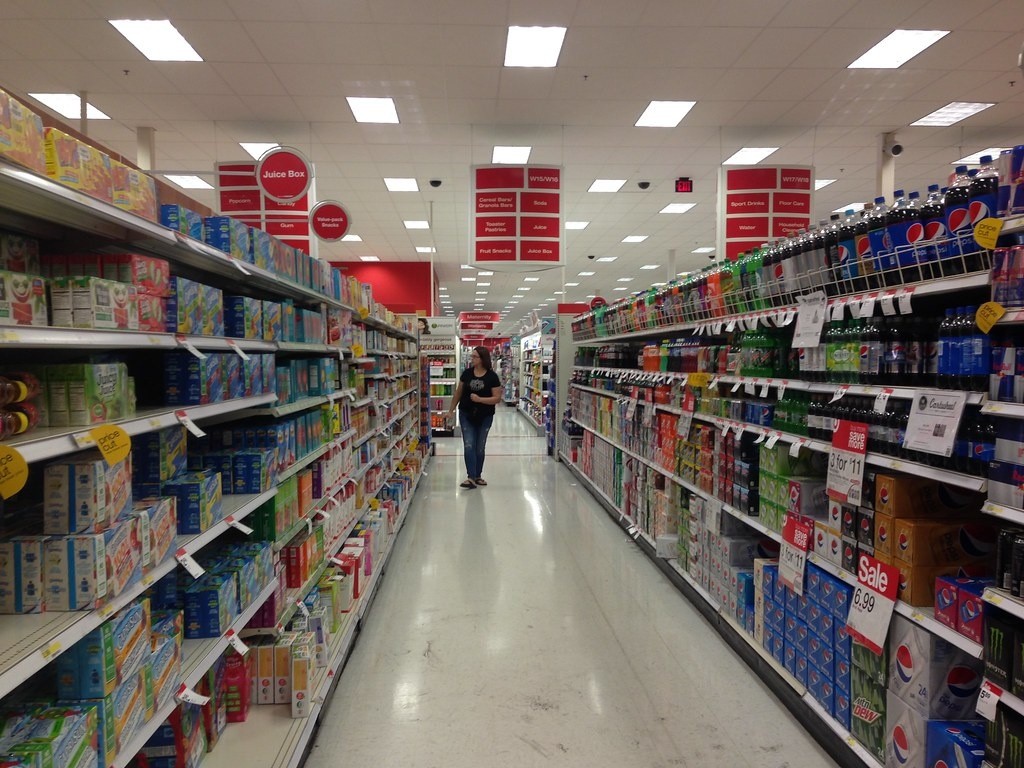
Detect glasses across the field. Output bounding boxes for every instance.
[470,355,480,359]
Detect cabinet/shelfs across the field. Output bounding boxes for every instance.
[1,159,432,768]
[547,146,1024,768]
[418,315,556,438]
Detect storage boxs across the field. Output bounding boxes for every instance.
[0,89,433,767]
[564,388,1024,768]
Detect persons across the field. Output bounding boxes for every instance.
[418,318,431,334]
[442,346,501,488]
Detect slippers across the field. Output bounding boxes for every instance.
[460,479,477,488]
[474,478,487,485]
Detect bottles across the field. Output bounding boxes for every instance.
[570,151,1024,486]
